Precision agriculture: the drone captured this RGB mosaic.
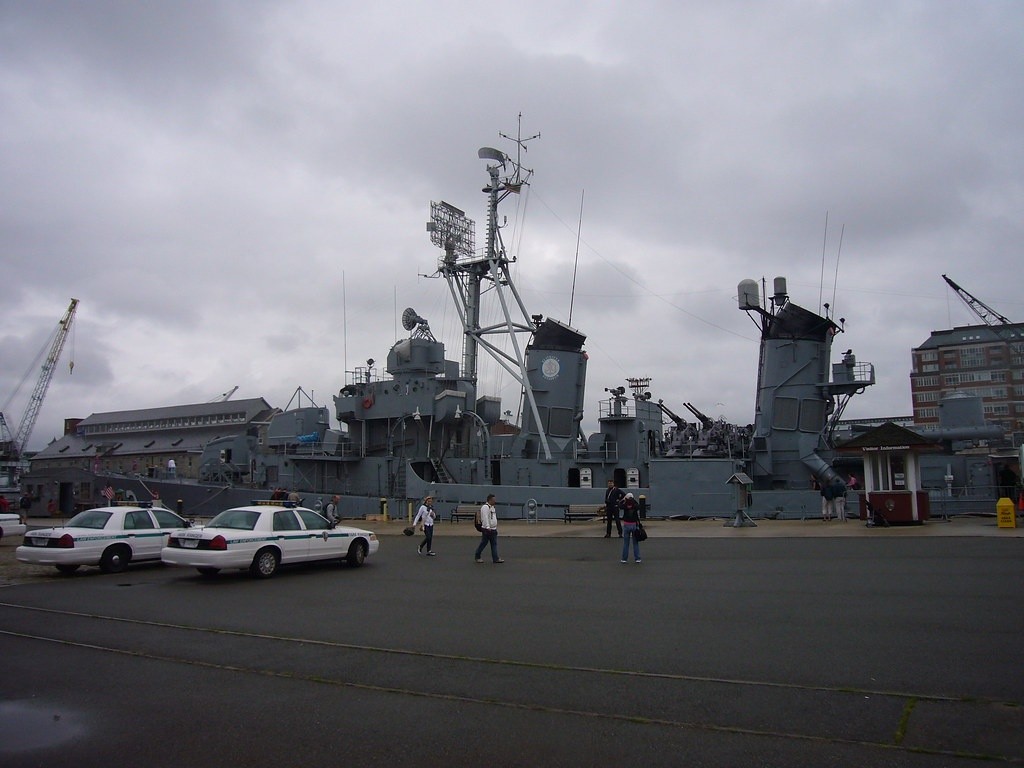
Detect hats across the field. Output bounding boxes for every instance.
[422,495,432,504]
[624,492,634,500]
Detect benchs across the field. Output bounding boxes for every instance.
[564,505,606,522]
[450,505,482,522]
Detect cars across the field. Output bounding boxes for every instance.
[160,499,380,579]
[15,499,206,573]
[0,514,27,538]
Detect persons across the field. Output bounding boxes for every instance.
[999,464,1016,496]
[274,488,300,502]
[475,495,504,562]
[19,492,31,526]
[315,496,323,515]
[833,478,847,522]
[604,480,625,537]
[168,459,176,478]
[811,473,821,490]
[0,496,9,513]
[48,499,55,512]
[821,479,833,521]
[412,497,436,556]
[619,492,642,563]
[846,473,857,490]
[326,496,340,523]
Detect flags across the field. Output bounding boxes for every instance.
[105,483,114,499]
[505,184,521,194]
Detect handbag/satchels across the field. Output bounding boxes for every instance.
[419,524,425,532]
[634,528,649,542]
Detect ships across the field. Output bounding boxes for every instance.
[89,112,1019,520]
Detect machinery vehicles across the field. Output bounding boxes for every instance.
[0,298,80,514]
[941,274,1024,359]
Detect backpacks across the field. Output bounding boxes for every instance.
[474,503,492,532]
[321,503,335,518]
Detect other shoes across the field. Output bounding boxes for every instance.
[619,534,623,539]
[416,542,423,555]
[822,518,832,522]
[493,558,504,563]
[636,558,642,563]
[604,533,612,538]
[426,550,436,556]
[620,558,627,563]
[475,556,484,563]
